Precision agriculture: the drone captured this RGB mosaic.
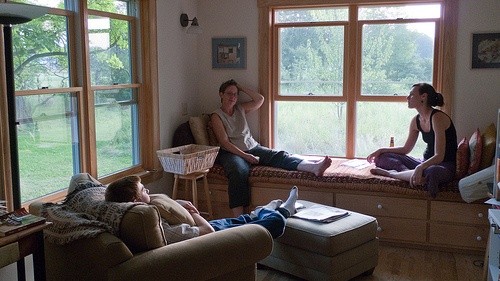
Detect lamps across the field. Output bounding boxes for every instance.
[181,14,204,34]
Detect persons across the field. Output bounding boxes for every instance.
[210,80,333,217]
[105,176,299,245]
[367,84,458,198]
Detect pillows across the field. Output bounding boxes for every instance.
[469,127,483,176]
[189,113,212,146]
[480,123,497,170]
[455,137,469,177]
[148,193,199,227]
[207,120,220,146]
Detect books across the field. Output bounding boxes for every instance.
[0,207,48,237]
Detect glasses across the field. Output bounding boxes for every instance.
[224,92,239,98]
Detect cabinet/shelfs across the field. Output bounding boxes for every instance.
[172,157,496,257]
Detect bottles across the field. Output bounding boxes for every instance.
[389,137,394,146]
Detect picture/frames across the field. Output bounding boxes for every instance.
[212,38,247,69]
[470,31,500,70]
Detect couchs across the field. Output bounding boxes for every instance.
[30,172,273,281]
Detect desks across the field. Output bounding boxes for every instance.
[0,222,51,281]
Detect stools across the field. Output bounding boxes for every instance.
[172,169,214,221]
[257,200,380,281]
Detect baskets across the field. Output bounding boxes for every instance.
[155,145,219,175]
